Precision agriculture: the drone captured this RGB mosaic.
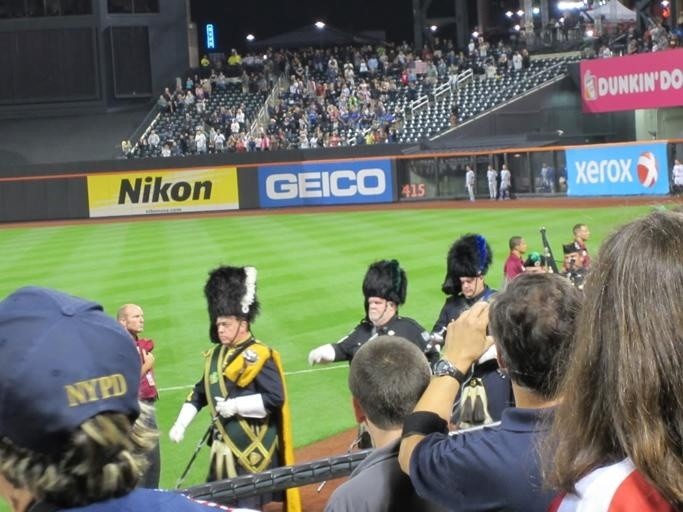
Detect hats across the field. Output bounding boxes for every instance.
[1,287,140,455]
[204,266,256,317]
[363,260,407,303]
[447,235,492,277]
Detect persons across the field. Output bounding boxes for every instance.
[0,207,682,512]
[499,164,512,200]
[120,7,683,158]
[465,165,476,201]
[486,165,498,200]
[671,159,683,194]
[541,162,567,193]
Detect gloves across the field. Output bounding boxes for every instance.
[170,402,196,442]
[215,394,266,419]
[309,344,336,366]
